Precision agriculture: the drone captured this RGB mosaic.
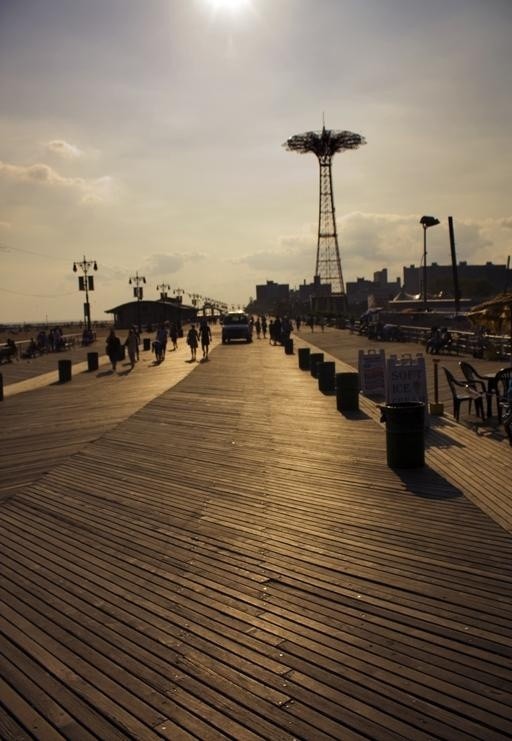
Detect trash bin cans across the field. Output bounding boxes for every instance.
[299,348,335,395]
[336,373,362,411]
[285,339,293,354]
[88,352,98,370]
[144,338,150,351]
[0,372,3,402]
[381,402,426,469]
[59,360,71,382]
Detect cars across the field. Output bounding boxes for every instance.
[220,312,252,343]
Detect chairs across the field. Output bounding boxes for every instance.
[441,361,511,425]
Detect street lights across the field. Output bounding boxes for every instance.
[73,255,97,342]
[419,215,441,309]
[128,271,146,334]
[157,283,233,310]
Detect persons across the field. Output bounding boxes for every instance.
[349,315,355,334]
[151,317,212,362]
[3,338,16,362]
[254,314,314,346]
[25,325,68,357]
[425,325,449,355]
[105,325,141,371]
[81,329,93,347]
